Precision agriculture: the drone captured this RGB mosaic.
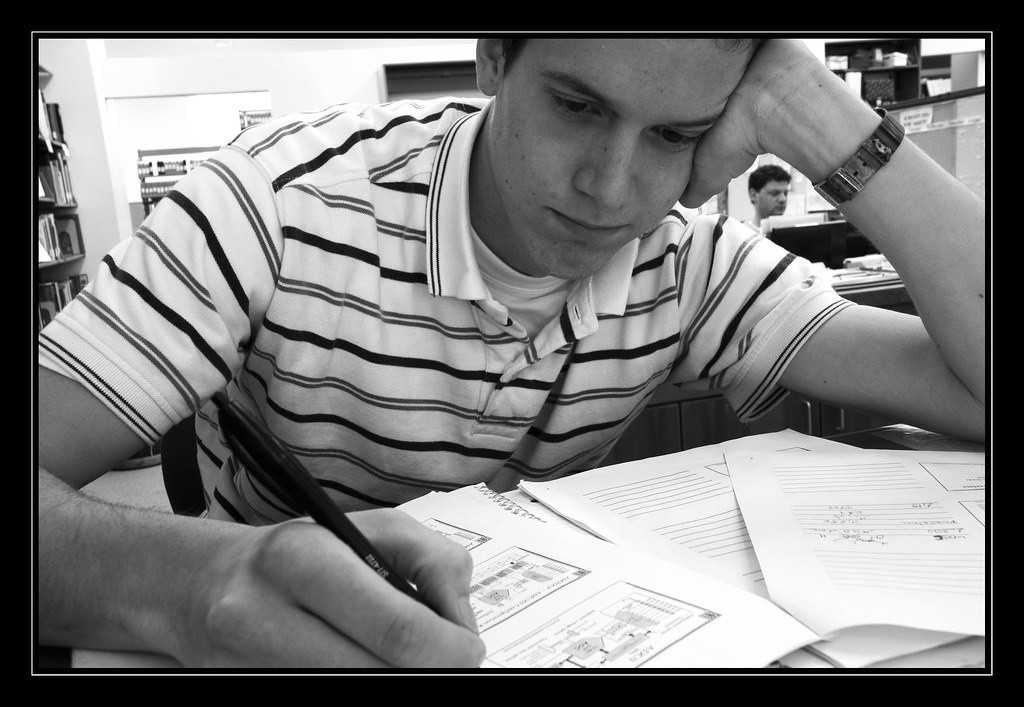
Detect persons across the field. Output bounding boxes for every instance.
[742,165,792,233]
[39,39,985,668]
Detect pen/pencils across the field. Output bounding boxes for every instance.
[210,384,434,611]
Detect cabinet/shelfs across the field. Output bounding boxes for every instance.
[35,101,85,270]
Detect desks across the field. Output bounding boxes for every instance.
[50,285,986,666]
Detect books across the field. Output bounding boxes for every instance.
[39,90,88,332]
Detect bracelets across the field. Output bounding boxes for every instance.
[813,106,905,206]
